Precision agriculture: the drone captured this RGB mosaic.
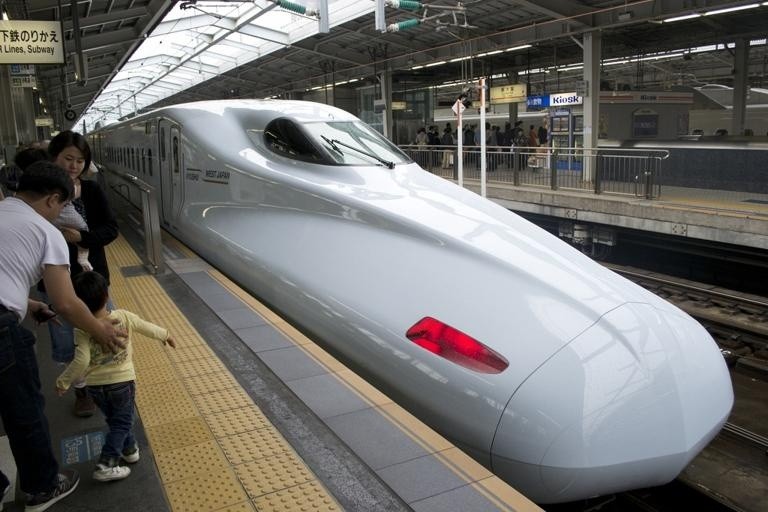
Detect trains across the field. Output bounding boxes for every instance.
[81,99,733,504]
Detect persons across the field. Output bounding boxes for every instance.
[415,123,548,172]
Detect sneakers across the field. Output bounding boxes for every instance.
[120,441,140,464]
[93,464,130,482]
[23,469,80,511]
[74,394,96,417]
